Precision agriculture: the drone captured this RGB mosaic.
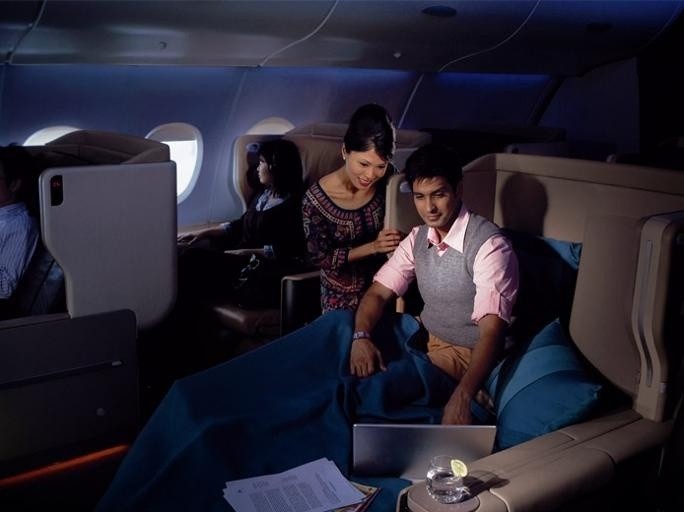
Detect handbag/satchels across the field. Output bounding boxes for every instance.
[233,254,280,310]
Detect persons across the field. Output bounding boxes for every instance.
[0,139,45,323]
[344,144,525,428]
[300,106,405,317]
[174,136,313,323]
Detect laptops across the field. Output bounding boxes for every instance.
[350,424,497,485]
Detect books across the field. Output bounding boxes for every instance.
[327,480,382,512]
[395,483,415,512]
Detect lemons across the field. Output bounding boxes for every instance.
[450,459,468,478]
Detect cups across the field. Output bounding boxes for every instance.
[426,456,464,504]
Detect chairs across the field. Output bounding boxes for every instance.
[2,130,177,479]
[192,124,427,350]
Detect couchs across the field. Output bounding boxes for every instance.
[384,153,684,511]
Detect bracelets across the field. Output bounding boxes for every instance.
[349,329,372,341]
